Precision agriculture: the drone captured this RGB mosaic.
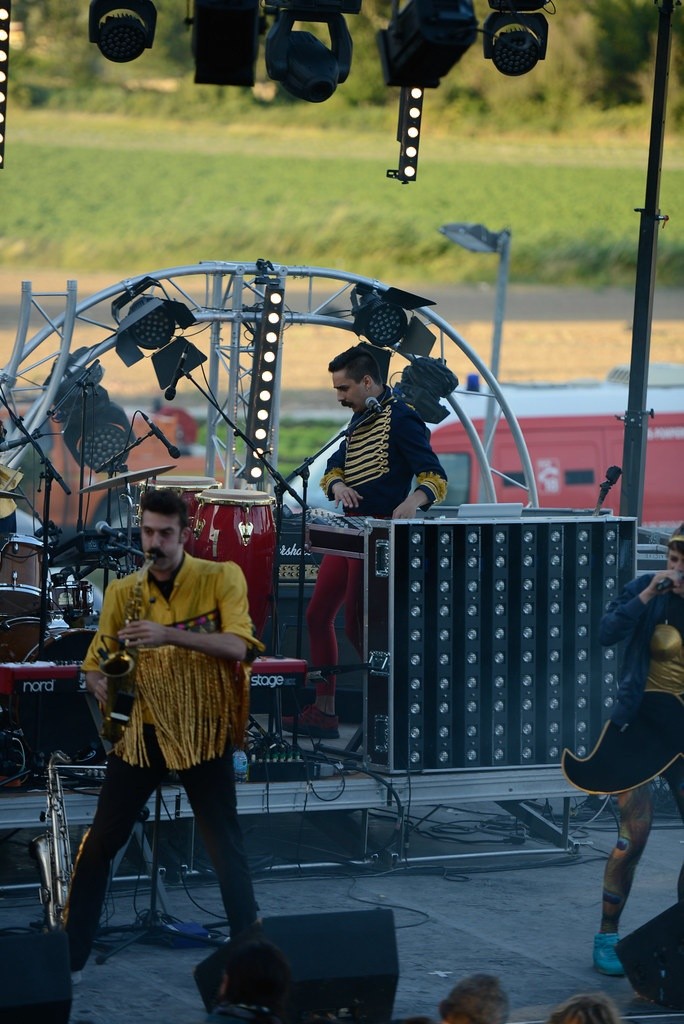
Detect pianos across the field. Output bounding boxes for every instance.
[0,661,310,789]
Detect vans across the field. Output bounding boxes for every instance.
[285,364,684,526]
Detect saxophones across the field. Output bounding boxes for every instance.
[98,552,159,742]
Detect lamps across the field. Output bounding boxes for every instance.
[88,0,555,102]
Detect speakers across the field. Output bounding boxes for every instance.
[614,897,684,1009]
[0,931,72,1024]
[196,909,400,1024]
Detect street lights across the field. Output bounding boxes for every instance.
[438,221,511,501]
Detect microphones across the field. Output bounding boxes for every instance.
[366,397,383,414]
[164,345,188,400]
[34,520,53,538]
[95,521,124,539]
[0,434,43,452]
[141,413,180,459]
[656,572,684,591]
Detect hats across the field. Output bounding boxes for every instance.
[668,524,684,542]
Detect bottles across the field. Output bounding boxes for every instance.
[232,747,248,785]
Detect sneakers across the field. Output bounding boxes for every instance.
[593,933,626,975]
[282,704,340,738]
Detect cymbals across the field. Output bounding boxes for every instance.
[78,464,178,494]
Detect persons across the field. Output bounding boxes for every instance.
[282,347,449,739]
[65,490,265,974]
[545,991,623,1024]
[202,937,288,1024]
[439,975,509,1024]
[562,524,684,977]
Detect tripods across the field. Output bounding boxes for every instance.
[92,785,229,966]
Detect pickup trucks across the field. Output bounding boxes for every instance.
[0,397,244,527]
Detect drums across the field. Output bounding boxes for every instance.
[0,533,52,617]
[133,475,222,575]
[52,580,95,619]
[187,489,276,648]
[0,617,104,669]
